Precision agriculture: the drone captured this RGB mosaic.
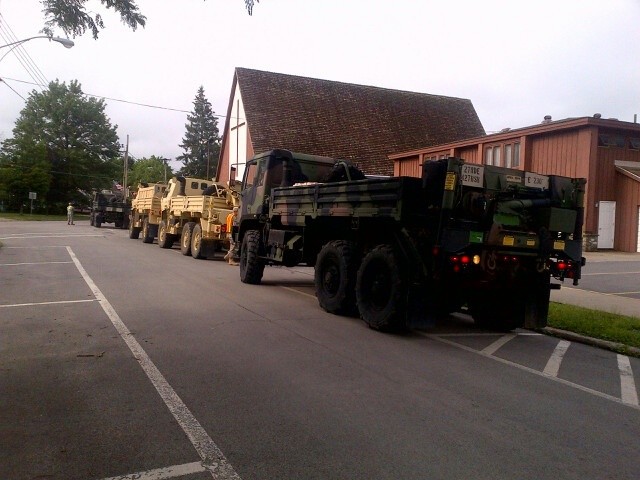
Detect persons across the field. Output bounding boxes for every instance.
[66,201,76,224]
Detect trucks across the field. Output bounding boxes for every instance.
[229,149,586,332]
[90,188,132,229]
[129,181,167,243]
[158,177,239,259]
